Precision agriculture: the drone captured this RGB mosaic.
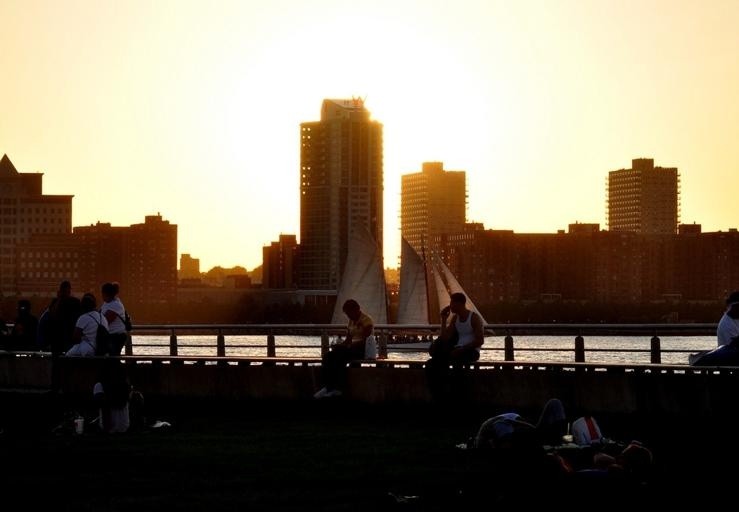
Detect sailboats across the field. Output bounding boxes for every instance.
[329,215,495,352]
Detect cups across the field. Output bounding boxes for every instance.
[74,418,84,433]
[562,434,573,445]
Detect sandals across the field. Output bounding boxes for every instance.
[109,424,129,434]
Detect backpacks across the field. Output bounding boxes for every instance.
[97,325,111,355]
[125,313,131,331]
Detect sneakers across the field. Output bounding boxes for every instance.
[314,387,341,399]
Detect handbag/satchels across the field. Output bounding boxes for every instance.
[689,345,736,366]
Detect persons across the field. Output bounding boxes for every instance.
[88,382,157,442]
[717,292,739,347]
[1,280,132,358]
[313,299,374,399]
[424,293,484,369]
